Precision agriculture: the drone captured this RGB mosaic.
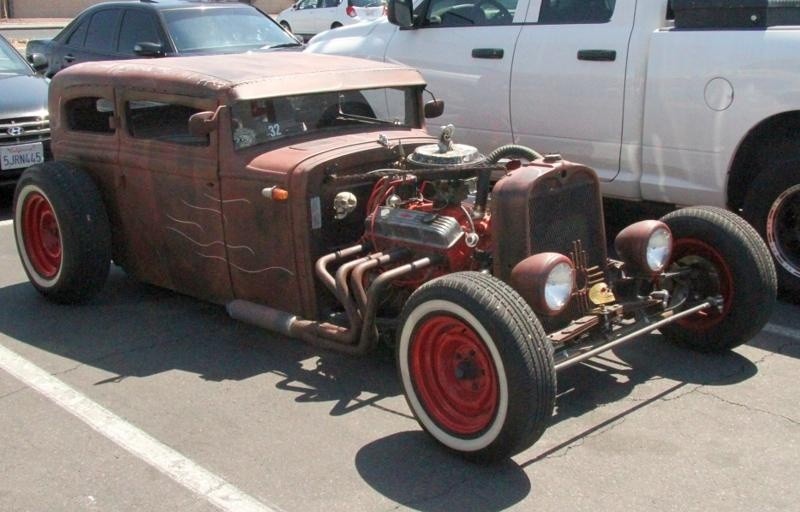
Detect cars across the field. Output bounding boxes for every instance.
[274,0,388,40]
[1,36,54,187]
[12,50,779,464]
[25,1,308,79]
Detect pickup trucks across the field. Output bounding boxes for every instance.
[302,1,799,311]
